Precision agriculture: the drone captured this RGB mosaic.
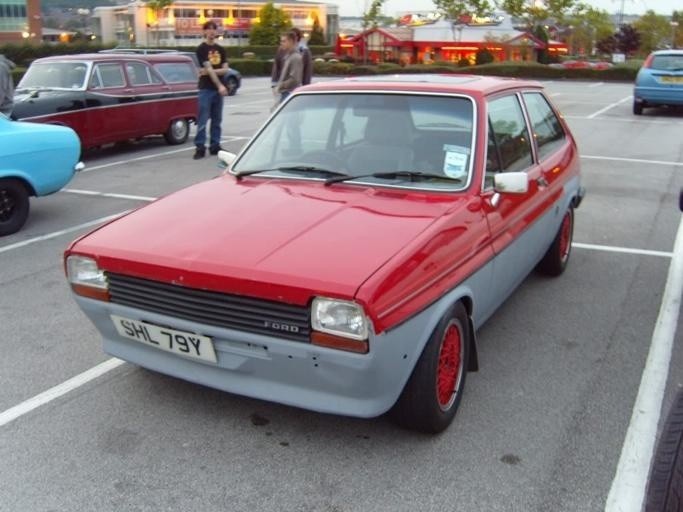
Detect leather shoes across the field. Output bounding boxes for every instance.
[193,145,222,159]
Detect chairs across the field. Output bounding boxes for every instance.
[349,109,417,178]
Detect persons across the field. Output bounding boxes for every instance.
[274,31,304,159]
[268,27,313,125]
[191,18,230,161]
[0,54,18,119]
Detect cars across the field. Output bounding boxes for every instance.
[633,49,683,114]
[64,73,586,435]
